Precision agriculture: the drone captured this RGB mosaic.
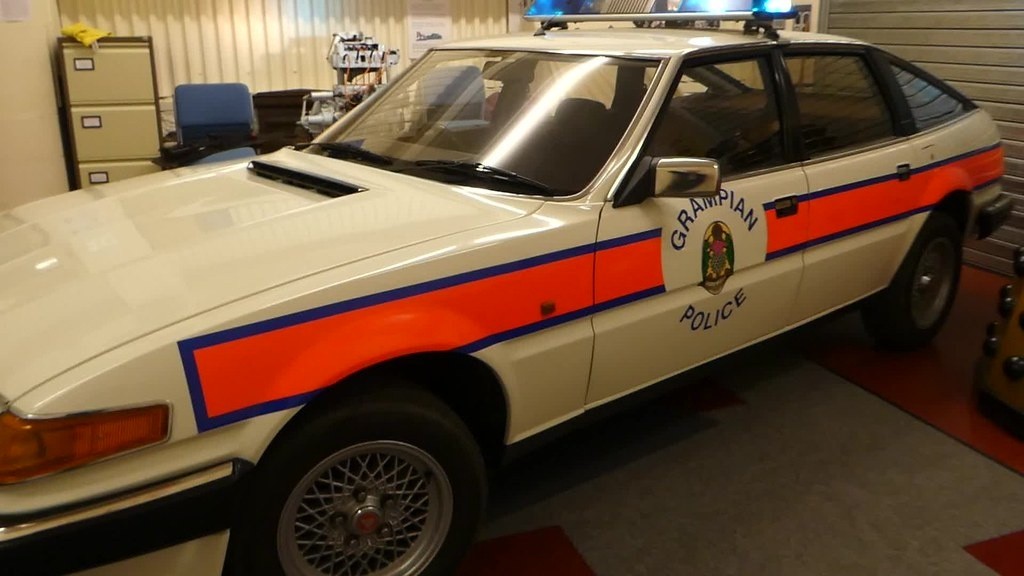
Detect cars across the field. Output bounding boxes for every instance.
[0,1,1013,576]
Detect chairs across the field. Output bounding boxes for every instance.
[538,98,606,199]
[153,82,332,172]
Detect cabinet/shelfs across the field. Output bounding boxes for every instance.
[57,36,166,189]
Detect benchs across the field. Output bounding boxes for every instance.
[674,105,882,168]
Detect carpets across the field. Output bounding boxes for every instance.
[780,260,1024,481]
